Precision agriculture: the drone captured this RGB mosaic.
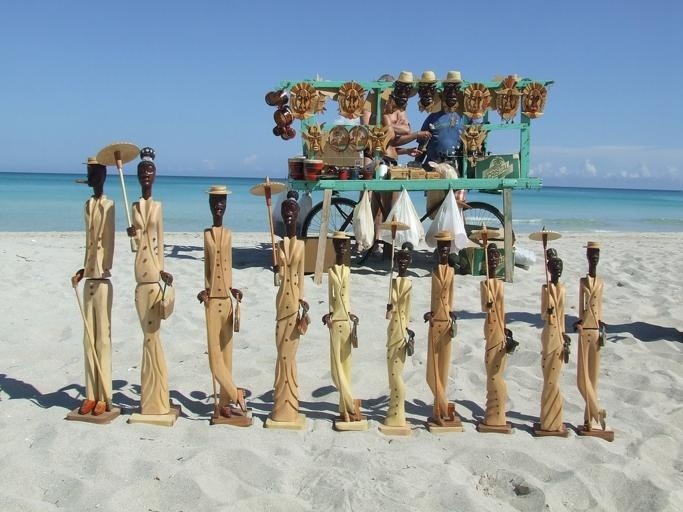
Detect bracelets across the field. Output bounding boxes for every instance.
[416,129,420,142]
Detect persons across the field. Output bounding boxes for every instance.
[416,111,475,219]
[360,74,431,254]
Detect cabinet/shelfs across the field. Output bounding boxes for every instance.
[280,80,555,283]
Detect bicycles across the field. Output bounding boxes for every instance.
[299,151,516,270]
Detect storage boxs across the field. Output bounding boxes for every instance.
[460,247,515,277]
[315,130,364,168]
[390,165,440,179]
[474,154,520,179]
[278,239,337,274]
